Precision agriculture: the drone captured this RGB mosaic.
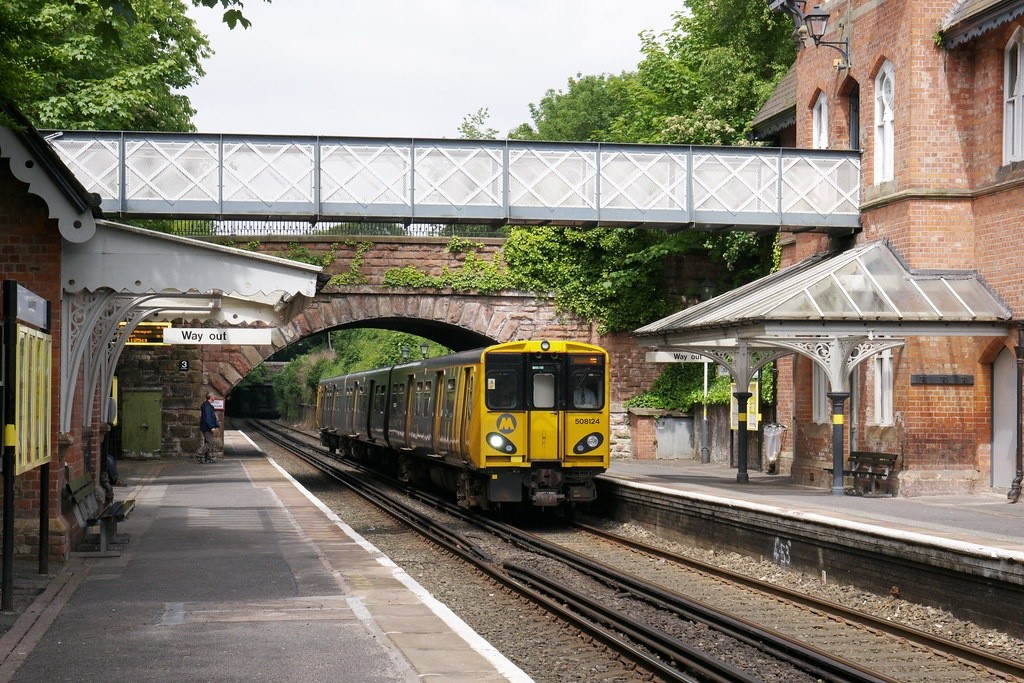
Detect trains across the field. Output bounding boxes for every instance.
[317,339,613,520]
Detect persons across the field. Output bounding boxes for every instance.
[193,392,219,463]
[574,375,598,407]
[107,455,127,486]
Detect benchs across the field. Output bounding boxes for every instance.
[823,450,899,497]
[66,475,136,558]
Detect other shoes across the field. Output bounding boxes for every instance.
[204,458,218,464]
[115,480,127,486]
[195,453,205,464]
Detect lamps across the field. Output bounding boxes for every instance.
[801,4,852,66]
